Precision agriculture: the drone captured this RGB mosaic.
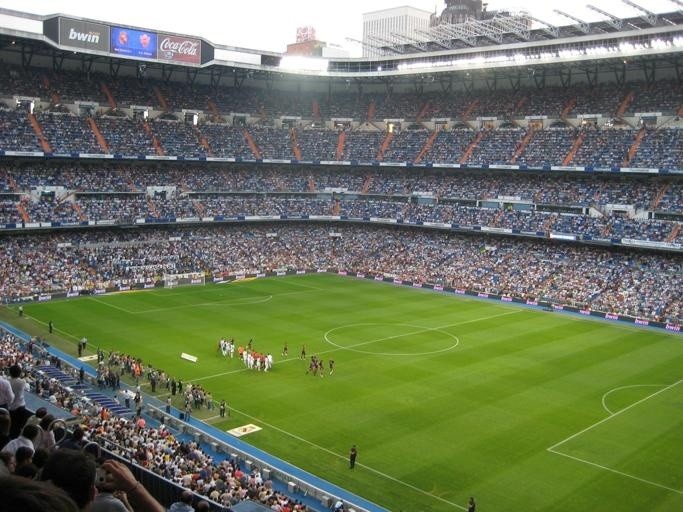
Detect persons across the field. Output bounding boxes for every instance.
[350,445,356,469]
[305,356,334,377]
[0,66,682,332]
[468,497,475,511]
[335,500,344,511]
[1,332,307,511]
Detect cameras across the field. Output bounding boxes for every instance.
[94,467,114,493]
